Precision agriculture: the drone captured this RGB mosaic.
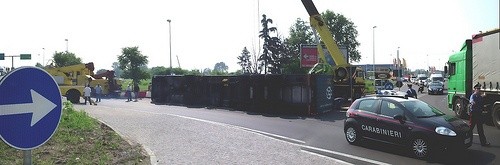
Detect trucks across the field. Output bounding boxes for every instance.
[445,29,500,128]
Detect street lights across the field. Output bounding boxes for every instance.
[373,26,377,76]
[43,48,45,68]
[65,39,68,64]
[168,19,171,76]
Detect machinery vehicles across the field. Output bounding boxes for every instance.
[45,63,115,104]
[301,0,369,86]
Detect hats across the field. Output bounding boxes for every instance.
[474,83,481,89]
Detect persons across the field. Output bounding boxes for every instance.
[126,84,132,102]
[148,83,152,103]
[83,83,92,105]
[470,84,490,146]
[418,80,424,92]
[134,84,139,99]
[95,84,101,102]
[406,84,417,99]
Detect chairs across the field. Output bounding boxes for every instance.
[382,102,388,114]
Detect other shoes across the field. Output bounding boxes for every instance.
[481,143,490,145]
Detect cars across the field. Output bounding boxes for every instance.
[375,79,404,99]
[401,74,450,94]
[344,94,474,160]
